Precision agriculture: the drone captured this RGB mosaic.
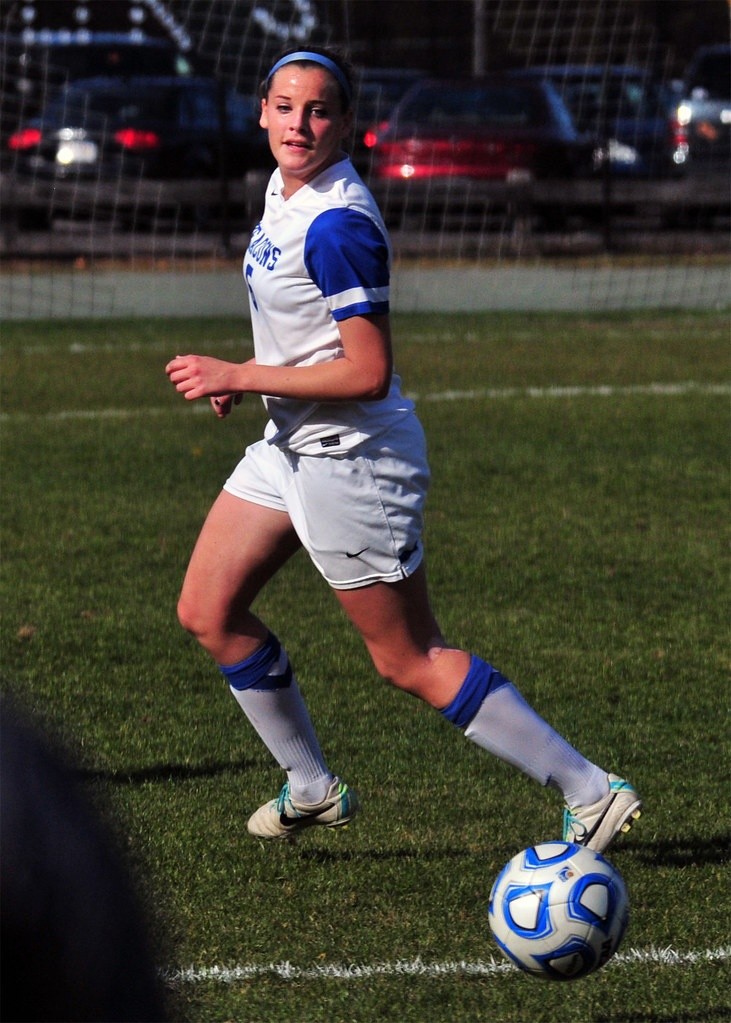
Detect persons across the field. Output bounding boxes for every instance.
[164,56,644,860]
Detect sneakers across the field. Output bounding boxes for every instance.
[563,774,642,852]
[247,777,357,841]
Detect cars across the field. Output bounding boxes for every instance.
[336,38,731,241]
[1,73,277,248]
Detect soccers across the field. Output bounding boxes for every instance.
[488,840,630,982]
